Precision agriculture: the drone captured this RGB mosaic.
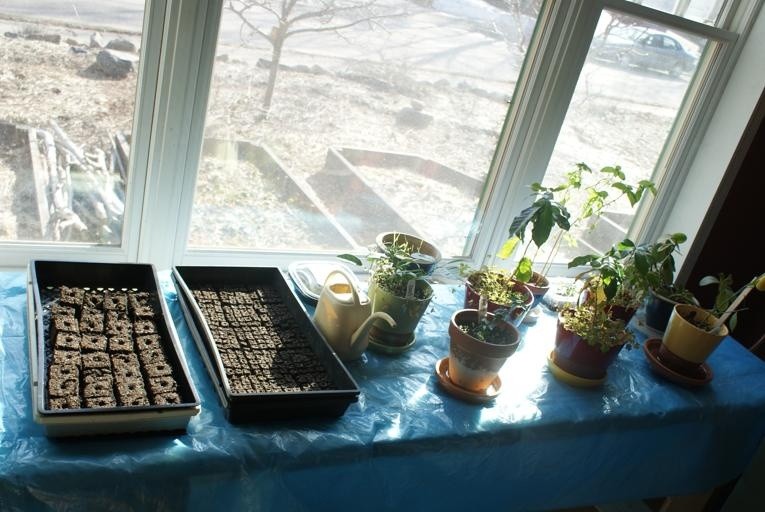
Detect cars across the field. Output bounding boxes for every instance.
[592,27,698,80]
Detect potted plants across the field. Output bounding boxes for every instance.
[434,286,521,402]
[644,273,701,333]
[642,278,729,386]
[463,180,570,329]
[577,233,685,329]
[508,163,657,323]
[368,233,435,352]
[547,244,646,390]
[374,230,442,278]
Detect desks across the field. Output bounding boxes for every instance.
[1,264,765,510]
